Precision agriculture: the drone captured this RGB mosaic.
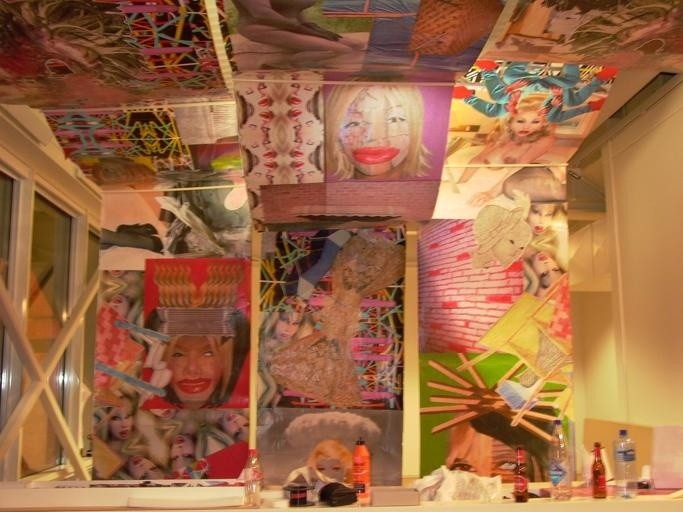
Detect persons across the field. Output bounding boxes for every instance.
[273,312,305,344]
[458,105,567,298]
[235,71,323,190]
[325,85,431,179]
[94,333,249,480]
[309,440,352,481]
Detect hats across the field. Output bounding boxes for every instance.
[503,162,566,201]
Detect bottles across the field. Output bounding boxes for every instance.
[288,484,307,507]
[352,437,370,498]
[614,430,638,499]
[512,444,529,502]
[547,420,573,500]
[591,442,607,498]
[244,449,263,510]
